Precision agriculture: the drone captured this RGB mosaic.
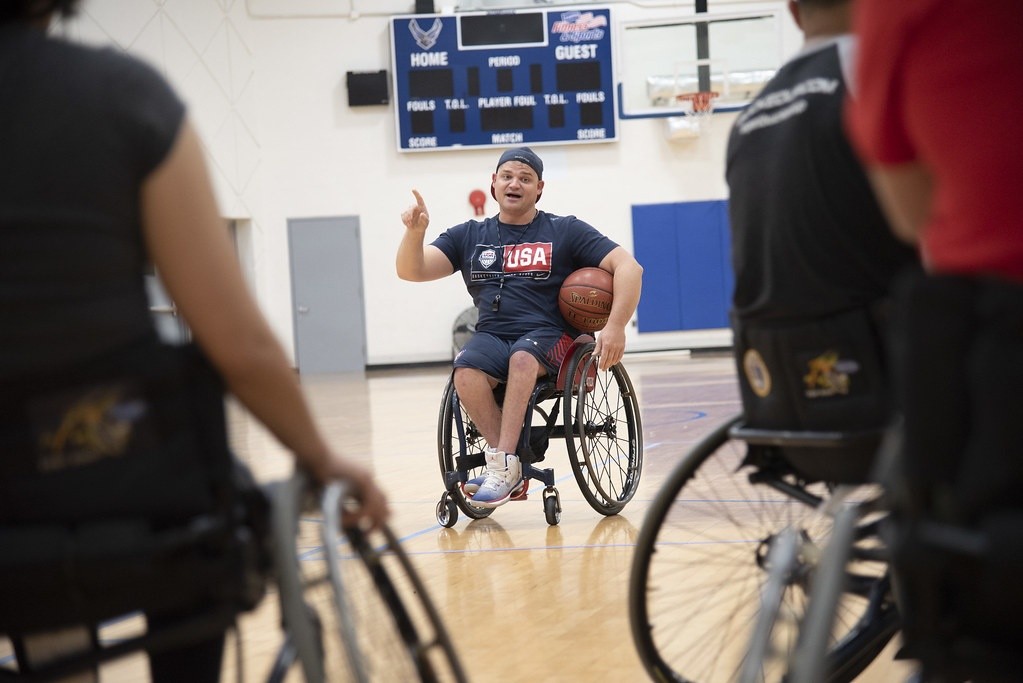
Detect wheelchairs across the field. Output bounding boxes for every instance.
[437,330,644,524]
[623,269,907,683]
[1,344,467,683]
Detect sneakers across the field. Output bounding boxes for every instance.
[463,448,495,492]
[470,448,525,509]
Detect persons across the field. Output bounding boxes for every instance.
[724,0,1023,683]
[0,0,391,682]
[397,147,644,507]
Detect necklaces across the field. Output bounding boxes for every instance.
[492,209,538,312]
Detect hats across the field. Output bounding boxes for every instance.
[491,147,544,203]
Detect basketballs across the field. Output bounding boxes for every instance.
[558,266,617,333]
